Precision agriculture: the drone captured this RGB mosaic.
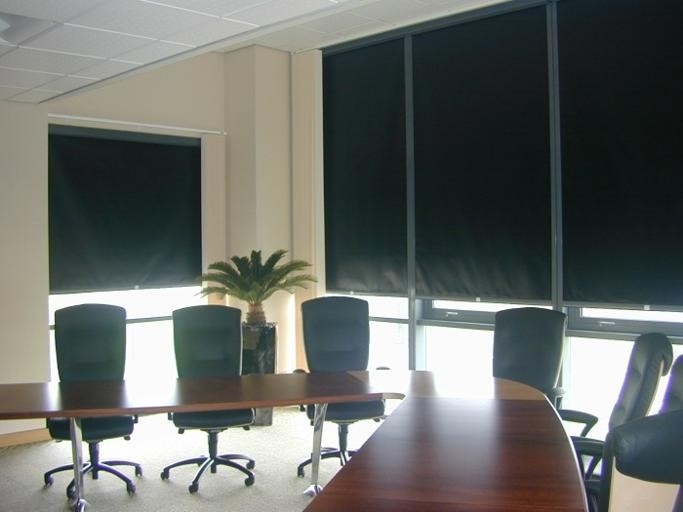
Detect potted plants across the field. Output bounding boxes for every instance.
[194,249,318,426]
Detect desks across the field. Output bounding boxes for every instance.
[1,370,591,511]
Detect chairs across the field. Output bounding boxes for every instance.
[555,333,673,480]
[45,304,141,497]
[293,296,384,478]
[160,304,257,493]
[599,409,683,511]
[567,354,683,511]
[493,307,568,409]
